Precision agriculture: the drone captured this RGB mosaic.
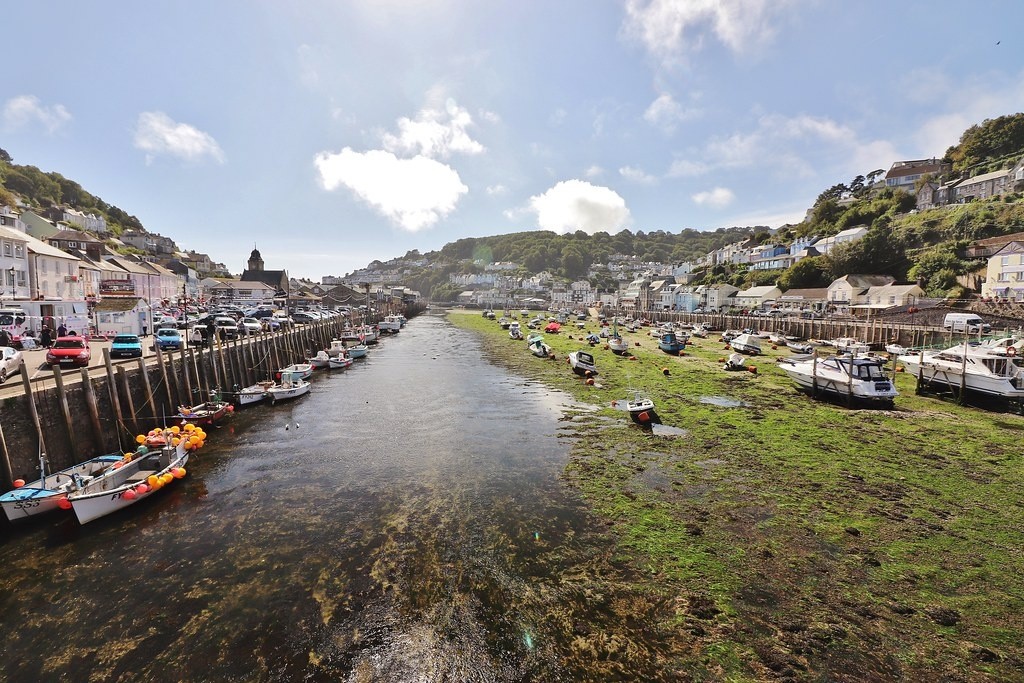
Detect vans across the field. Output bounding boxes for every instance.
[944,312,991,334]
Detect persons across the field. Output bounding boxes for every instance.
[192,318,268,352]
[139,318,149,338]
[20,323,67,349]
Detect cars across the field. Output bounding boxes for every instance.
[0,346,24,384]
[149,298,407,407]
[110,333,143,358]
[45,335,92,367]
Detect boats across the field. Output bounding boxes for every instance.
[66,437,192,527]
[171,367,230,431]
[624,373,658,424]
[0,434,126,522]
[479,291,1024,415]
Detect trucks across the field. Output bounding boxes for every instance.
[0,299,91,350]
